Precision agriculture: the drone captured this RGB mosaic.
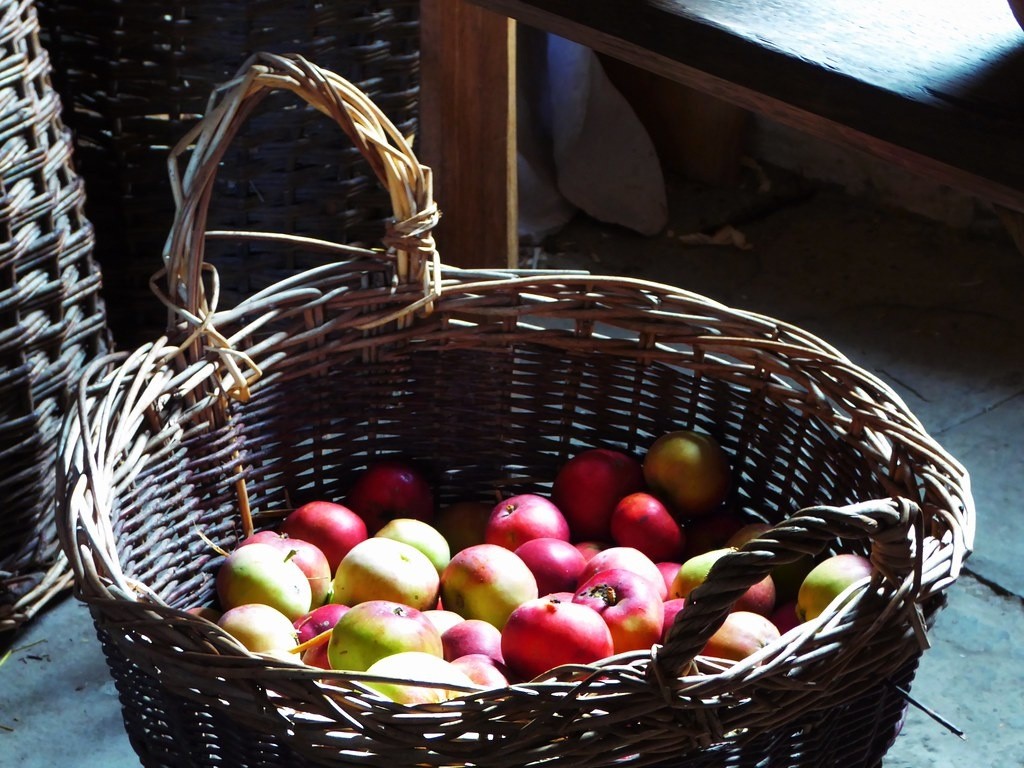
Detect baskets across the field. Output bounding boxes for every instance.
[41,0,420,332]
[56,50,975,768]
[0,0,117,631]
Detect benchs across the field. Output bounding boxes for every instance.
[416,0,1024,286]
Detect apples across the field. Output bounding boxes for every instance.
[184,434,873,702]
